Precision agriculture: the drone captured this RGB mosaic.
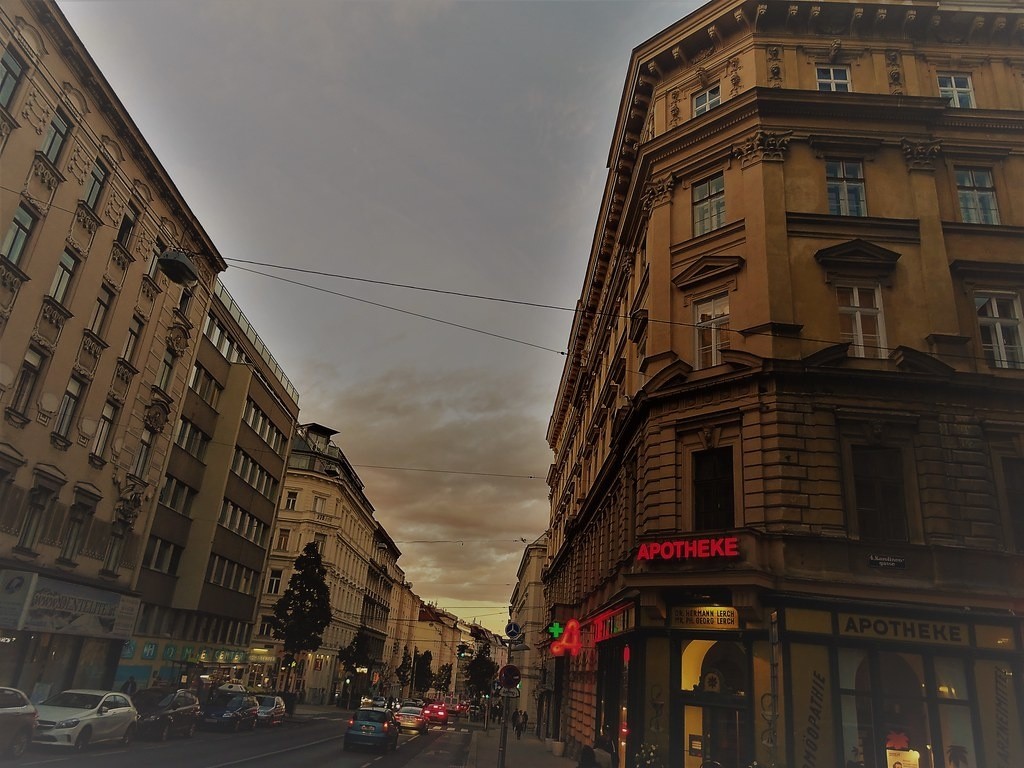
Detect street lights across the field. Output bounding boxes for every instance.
[497,644,531,768]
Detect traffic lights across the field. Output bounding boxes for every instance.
[485,693,489,699]
[457,644,466,657]
[345,676,350,685]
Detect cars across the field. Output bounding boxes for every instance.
[0,685,40,759]
[394,706,430,734]
[401,696,482,726]
[254,694,286,728]
[343,706,401,754]
[132,686,204,742]
[197,692,260,732]
[30,688,138,752]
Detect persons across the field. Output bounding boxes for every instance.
[489,699,503,725]
[511,708,529,740]
[121,676,136,696]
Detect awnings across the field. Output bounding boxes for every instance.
[171,659,250,668]
